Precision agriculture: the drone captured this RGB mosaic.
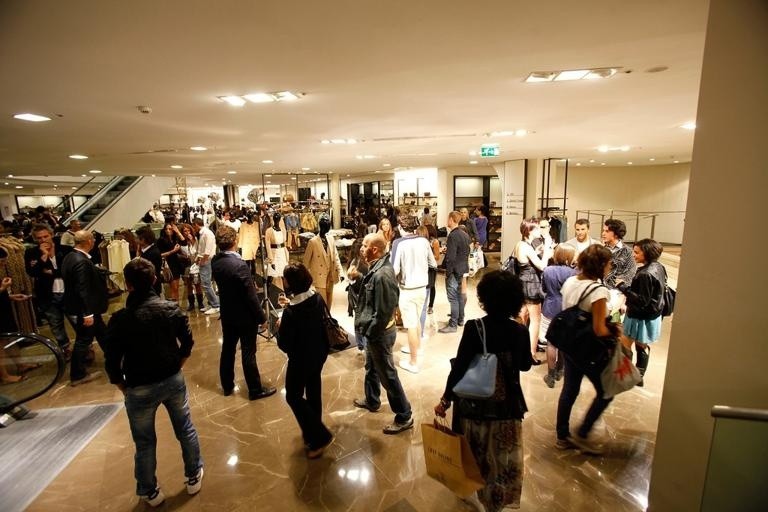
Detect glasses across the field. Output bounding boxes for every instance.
[538,224,553,231]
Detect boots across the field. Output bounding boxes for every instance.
[195,290,205,314]
[182,292,196,313]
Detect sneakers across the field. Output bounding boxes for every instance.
[60,347,74,363]
[351,396,380,415]
[427,308,435,315]
[543,367,555,389]
[399,345,427,357]
[142,482,166,508]
[198,304,222,319]
[397,359,422,375]
[437,323,458,335]
[302,436,339,461]
[457,319,465,327]
[85,344,97,363]
[555,361,565,382]
[181,464,208,496]
[551,432,608,457]
[380,415,416,438]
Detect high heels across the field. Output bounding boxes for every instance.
[531,357,542,367]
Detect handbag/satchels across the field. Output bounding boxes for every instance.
[183,266,202,287]
[420,412,490,501]
[321,316,352,352]
[468,248,487,275]
[597,344,646,405]
[448,349,498,400]
[660,284,676,319]
[544,303,615,363]
[157,267,175,284]
[498,255,521,277]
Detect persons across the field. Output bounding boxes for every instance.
[212,225,278,400]
[301,216,344,310]
[224,211,242,236]
[279,265,343,432]
[238,211,261,277]
[265,212,289,283]
[347,198,668,512]
[107,255,193,458]
[0,205,115,387]
[213,209,226,235]
[153,219,220,315]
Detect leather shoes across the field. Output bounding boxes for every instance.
[0,373,31,386]
[538,339,548,345]
[535,346,546,353]
[221,380,238,399]
[247,384,278,405]
[65,368,105,387]
[12,361,44,374]
